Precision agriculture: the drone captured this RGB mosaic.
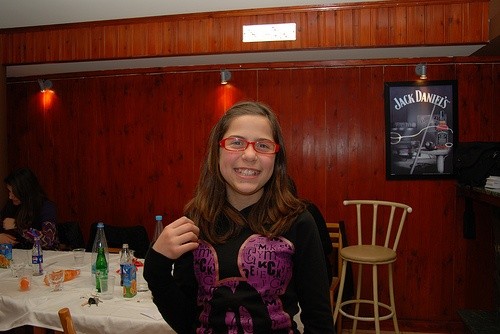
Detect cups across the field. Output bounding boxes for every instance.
[18,275,30,291]
[99,275,114,300]
[73,248,85,267]
[48,268,64,291]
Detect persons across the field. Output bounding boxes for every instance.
[0,170,65,251]
[142,101,336,333]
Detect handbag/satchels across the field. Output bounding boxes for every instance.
[452,142,500,186]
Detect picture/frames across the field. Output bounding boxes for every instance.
[383,79,461,182]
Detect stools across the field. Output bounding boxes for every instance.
[333,199,413,334]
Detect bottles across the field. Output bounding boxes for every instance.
[123,264,136,298]
[119,243,132,286]
[96,247,108,292]
[91,222,109,289]
[32,237,44,276]
[152,216,163,244]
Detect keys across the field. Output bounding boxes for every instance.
[82,296,104,307]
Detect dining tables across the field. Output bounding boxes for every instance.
[0,247,177,334]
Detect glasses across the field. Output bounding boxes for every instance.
[218,137,280,153]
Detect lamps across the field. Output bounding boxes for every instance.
[38,78,53,93]
[218,70,232,86]
[414,63,430,81]
